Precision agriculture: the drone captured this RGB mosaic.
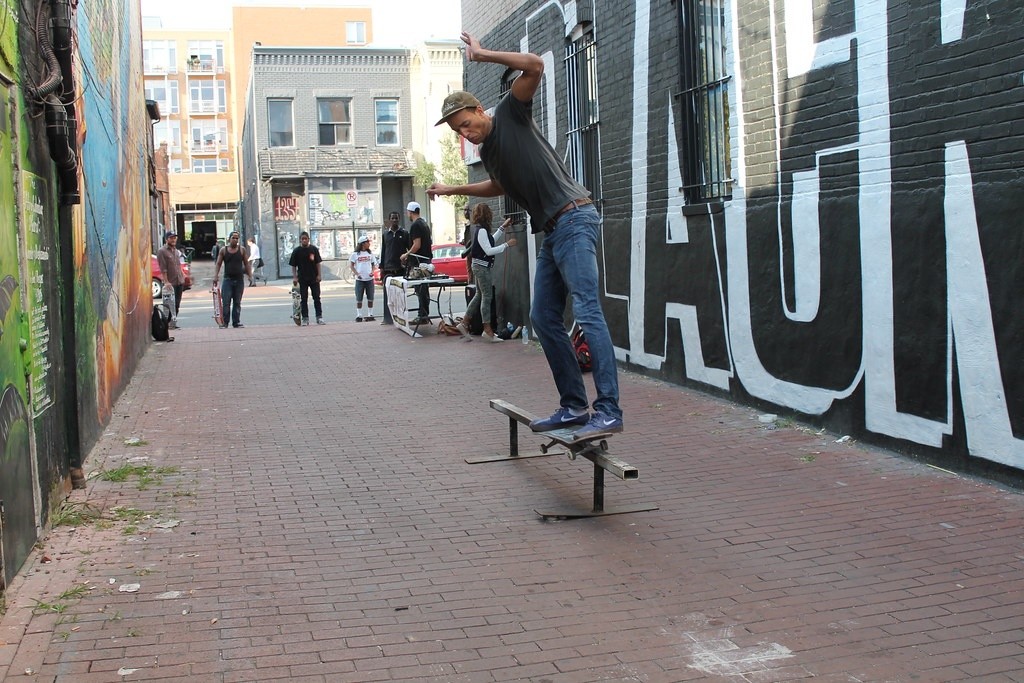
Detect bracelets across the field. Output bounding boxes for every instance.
[498,225,505,232]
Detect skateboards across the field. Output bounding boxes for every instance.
[209,282,224,326]
[162,286,177,329]
[529,418,614,461]
[289,283,301,326]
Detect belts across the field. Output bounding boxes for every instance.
[542,197,592,234]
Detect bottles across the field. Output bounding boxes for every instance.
[506,321,513,330]
[522,326,529,345]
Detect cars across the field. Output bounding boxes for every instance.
[374,242,470,286]
[151,254,191,298]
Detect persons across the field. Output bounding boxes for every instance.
[212,231,253,330]
[211,242,223,266]
[288,231,327,327]
[423,30,625,443]
[455,201,517,343]
[247,238,268,287]
[157,231,185,330]
[399,201,434,326]
[349,235,376,322]
[378,210,410,326]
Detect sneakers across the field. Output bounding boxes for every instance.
[529,406,591,432]
[301,316,310,325]
[573,411,623,439]
[317,319,326,325]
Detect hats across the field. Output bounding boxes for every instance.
[407,201,421,212]
[435,91,483,126]
[166,231,178,237]
[357,236,369,243]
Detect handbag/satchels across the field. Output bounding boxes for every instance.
[257,257,264,268]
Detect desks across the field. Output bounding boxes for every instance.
[386,275,455,337]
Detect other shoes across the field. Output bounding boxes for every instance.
[264,277,267,285]
[380,321,392,325]
[456,323,473,340]
[233,323,244,328]
[219,323,228,329]
[481,331,504,342]
[249,284,256,286]
[356,315,375,322]
[408,316,428,324]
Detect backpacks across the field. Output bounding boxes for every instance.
[572,329,592,372]
[152,303,172,341]
